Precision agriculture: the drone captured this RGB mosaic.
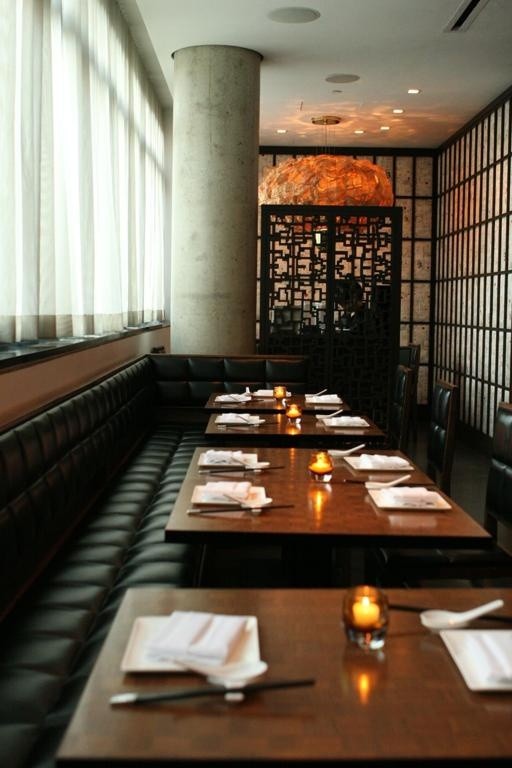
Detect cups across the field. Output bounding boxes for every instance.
[272,384,287,404]
[342,585,389,650]
[285,404,303,424]
[308,450,335,484]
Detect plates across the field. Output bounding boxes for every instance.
[215,415,261,427]
[321,417,369,428]
[366,486,452,512]
[191,484,268,509]
[305,393,343,405]
[439,631,510,691]
[123,614,262,677]
[345,457,413,471]
[198,453,259,467]
[215,395,251,402]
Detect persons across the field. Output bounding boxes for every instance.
[331,277,373,338]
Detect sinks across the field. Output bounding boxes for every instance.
[0,354,311,768]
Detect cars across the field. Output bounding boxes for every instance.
[274,386,389,651]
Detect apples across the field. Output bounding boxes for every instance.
[352,597,379,628]
[276,390,284,397]
[289,405,298,417]
[312,455,329,473]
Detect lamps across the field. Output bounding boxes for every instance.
[368,403,512,588]
[423,379,459,497]
[347,344,422,449]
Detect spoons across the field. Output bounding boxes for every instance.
[421,600,504,629]
[232,456,269,470]
[365,474,410,489]
[316,409,344,420]
[305,390,327,397]
[235,415,265,425]
[328,443,365,458]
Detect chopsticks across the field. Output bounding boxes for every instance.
[387,599,512,624]
[342,479,425,488]
[186,504,294,515]
[197,464,286,473]
[108,679,317,709]
